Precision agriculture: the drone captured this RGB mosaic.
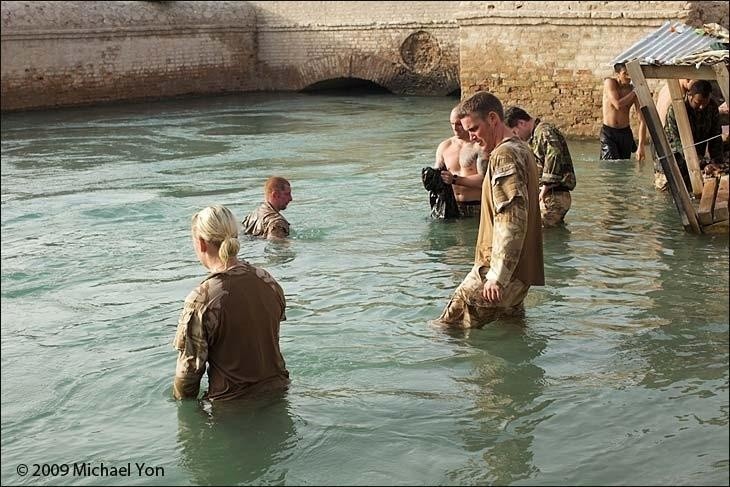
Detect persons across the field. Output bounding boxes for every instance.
[170,205,290,415]
[500,106,575,226]
[432,93,550,330]
[600,64,724,193]
[242,178,294,243]
[429,106,489,218]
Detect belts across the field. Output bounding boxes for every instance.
[553,186,569,192]
[456,200,480,204]
